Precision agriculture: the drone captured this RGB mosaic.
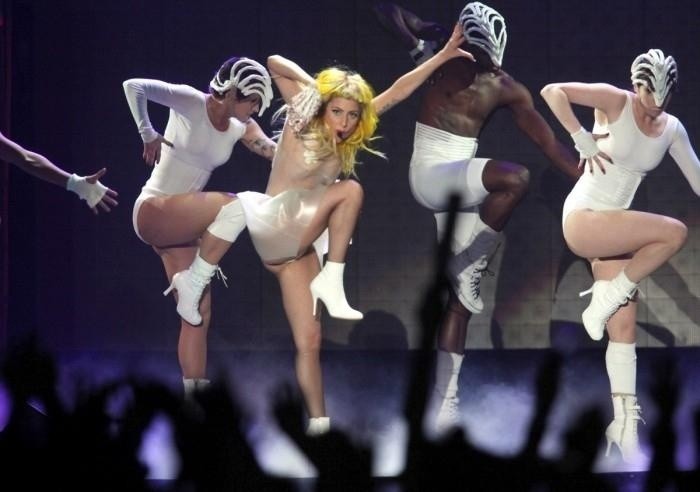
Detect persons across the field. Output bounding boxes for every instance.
[241,20,473,431]
[370,2,582,437]
[541,49,699,470]
[1,133,119,215]
[117,55,279,396]
[1,300,699,491]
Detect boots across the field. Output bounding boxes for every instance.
[310,261,364,320]
[445,250,504,314]
[579,269,645,341]
[164,248,229,325]
[605,396,650,466]
[422,388,465,433]
